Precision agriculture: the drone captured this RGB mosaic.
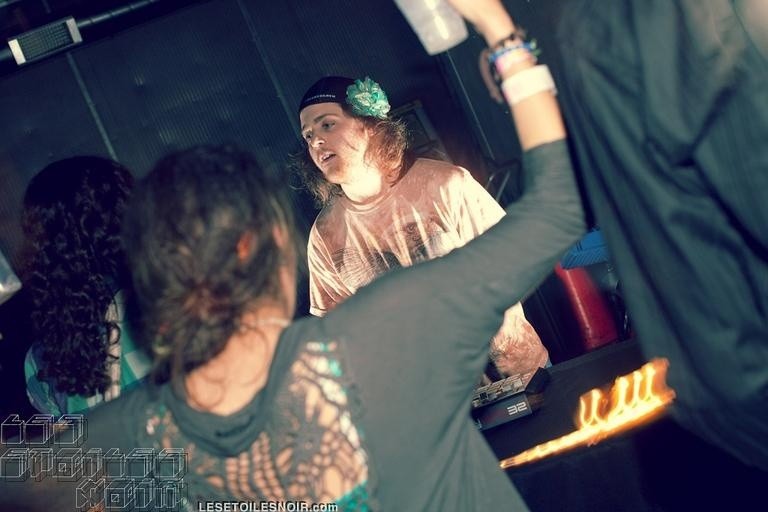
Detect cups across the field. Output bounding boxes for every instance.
[395,0,470,56]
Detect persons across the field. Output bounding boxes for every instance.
[549,2,767,481]
[0,2,586,512]
[19,153,154,422]
[297,73,552,391]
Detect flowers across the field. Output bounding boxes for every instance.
[346,79,393,120]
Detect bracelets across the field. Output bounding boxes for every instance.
[489,40,541,61]
[501,63,556,107]
[484,29,530,53]
